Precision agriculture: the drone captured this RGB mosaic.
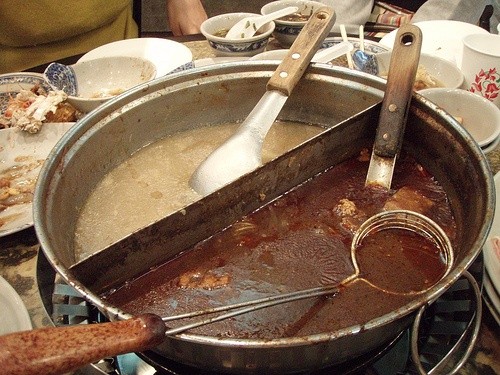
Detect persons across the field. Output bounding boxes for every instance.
[0,0,209,74]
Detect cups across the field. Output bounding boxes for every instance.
[460,34,499,109]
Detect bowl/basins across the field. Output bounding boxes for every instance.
[42,57,156,114]
[261,0,328,48]
[249,36,392,75]
[376,50,500,154]
[200,13,275,57]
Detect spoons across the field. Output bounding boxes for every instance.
[226,7,299,38]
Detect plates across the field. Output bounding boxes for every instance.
[75,37,193,80]
[0,276,32,336]
[0,122,76,236]
[1,72,53,127]
[379,19,490,68]
[483,170,499,325]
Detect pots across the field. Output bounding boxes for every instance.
[32,59,495,375]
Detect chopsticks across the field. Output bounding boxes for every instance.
[341,24,364,70]
